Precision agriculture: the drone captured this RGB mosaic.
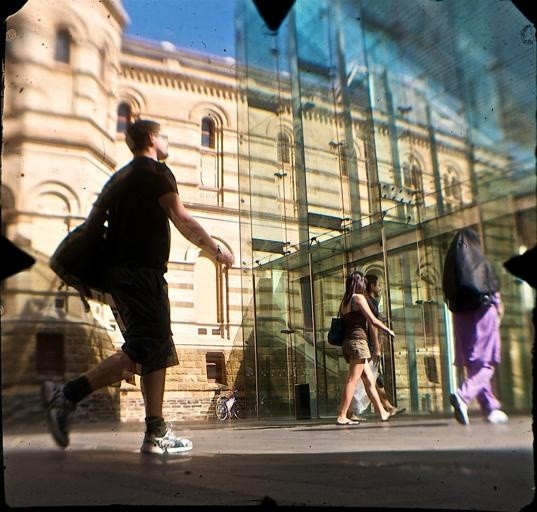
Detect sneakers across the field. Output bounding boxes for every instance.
[141,432,193,454]
[484,410,509,424]
[42,380,74,446]
[450,394,469,426]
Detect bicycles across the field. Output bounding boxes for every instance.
[215,388,245,421]
[250,393,273,417]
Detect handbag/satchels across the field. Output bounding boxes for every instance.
[328,318,344,346]
[50,221,112,304]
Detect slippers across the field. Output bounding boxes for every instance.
[337,408,406,424]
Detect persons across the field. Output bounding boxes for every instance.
[346,273,407,422]
[335,270,396,426]
[441,228,511,427]
[45,116,236,455]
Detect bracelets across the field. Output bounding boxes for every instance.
[383,327,390,335]
[214,244,224,261]
